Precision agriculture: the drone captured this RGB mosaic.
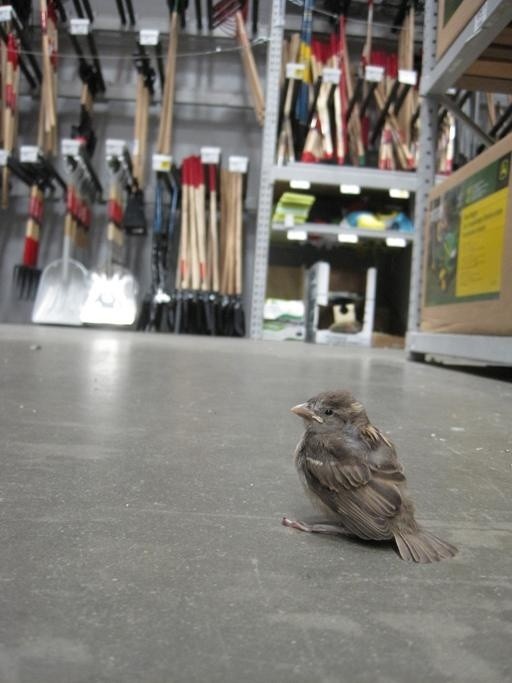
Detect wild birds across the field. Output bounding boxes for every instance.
[282,389,459,566]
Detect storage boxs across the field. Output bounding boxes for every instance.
[419,130,512,335]
[434,0,512,95]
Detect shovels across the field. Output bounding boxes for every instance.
[32,155,139,326]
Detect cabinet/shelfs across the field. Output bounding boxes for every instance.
[1,0,451,348]
[405,1,512,366]
